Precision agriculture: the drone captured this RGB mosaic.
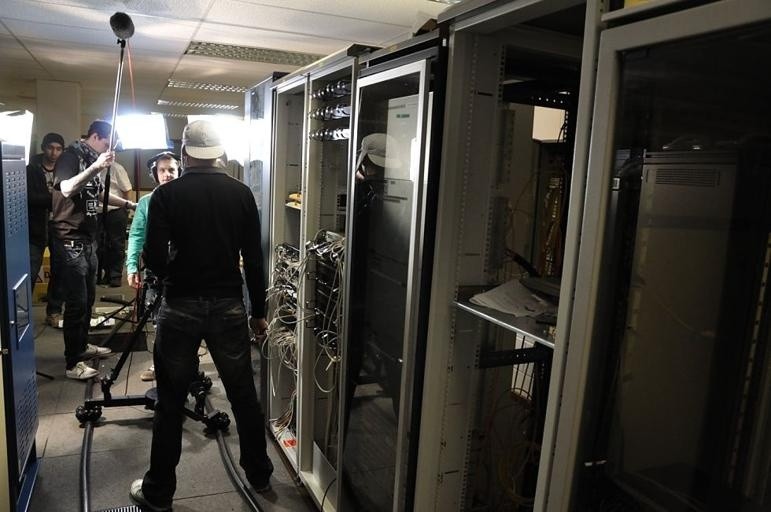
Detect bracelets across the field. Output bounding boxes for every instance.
[126,200,130,209]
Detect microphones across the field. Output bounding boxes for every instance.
[110,11,134,39]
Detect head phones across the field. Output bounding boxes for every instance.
[147,151,182,184]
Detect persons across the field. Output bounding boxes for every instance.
[27,132,66,328]
[96,161,134,287]
[129,119,275,512]
[343,133,414,452]
[51,118,138,381]
[126,153,180,289]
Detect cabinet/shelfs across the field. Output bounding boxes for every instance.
[242,4,770,512]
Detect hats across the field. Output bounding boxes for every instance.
[183,121,226,160]
[356,133,401,171]
[80,121,110,140]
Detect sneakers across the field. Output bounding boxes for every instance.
[109,277,121,287]
[141,365,156,381]
[65,361,99,380]
[130,478,171,512]
[79,343,111,359]
[256,483,273,493]
[43,314,63,328]
